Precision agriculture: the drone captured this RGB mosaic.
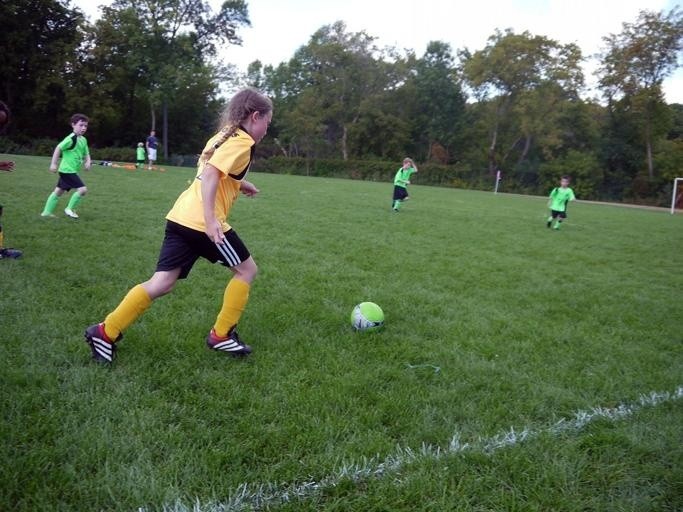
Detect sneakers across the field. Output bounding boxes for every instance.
[65,208,79,219]
[207,324,252,354]
[85,323,123,364]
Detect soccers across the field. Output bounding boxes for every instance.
[350,302,384,333]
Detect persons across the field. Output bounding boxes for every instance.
[392,157,419,212]
[546,176,576,230]
[146,130,159,169]
[0,103,24,258]
[41,114,91,217]
[136,142,146,168]
[85,89,274,364]
